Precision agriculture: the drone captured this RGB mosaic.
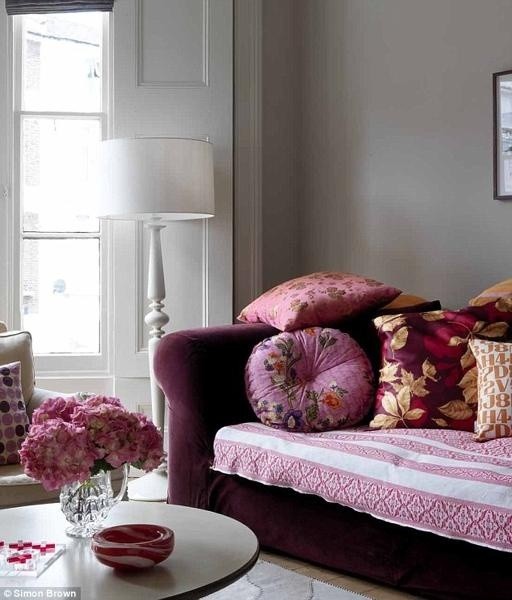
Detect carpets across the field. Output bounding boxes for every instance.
[200,559,372,600]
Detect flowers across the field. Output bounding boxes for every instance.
[14,392,168,522]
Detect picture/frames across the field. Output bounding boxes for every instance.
[492,71,512,201]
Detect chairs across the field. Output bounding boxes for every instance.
[0,322,129,508]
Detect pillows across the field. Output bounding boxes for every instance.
[0,360,31,465]
[236,270,510,447]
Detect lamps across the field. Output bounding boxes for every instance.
[96,137,214,498]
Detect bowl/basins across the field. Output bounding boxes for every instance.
[90,524,175,576]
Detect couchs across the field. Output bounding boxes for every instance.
[152,322,511,599]
